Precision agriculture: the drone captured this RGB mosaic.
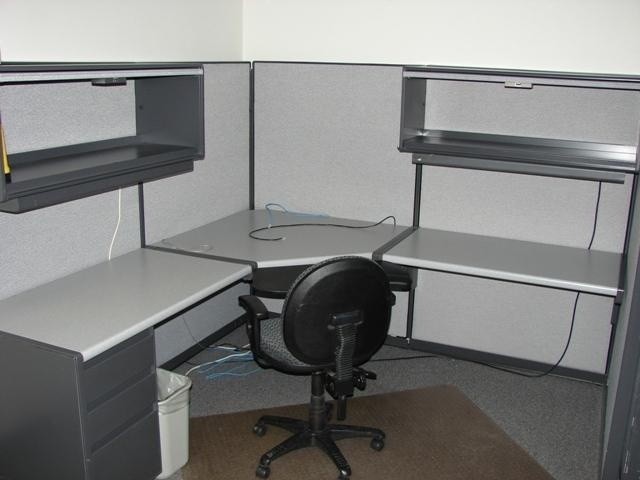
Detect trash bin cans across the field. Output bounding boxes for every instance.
[158,369,192,479]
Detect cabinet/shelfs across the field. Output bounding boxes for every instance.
[76,326,164,480]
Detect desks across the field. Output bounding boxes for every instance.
[380,228,626,307]
[0,242,253,479]
[150,206,417,270]
